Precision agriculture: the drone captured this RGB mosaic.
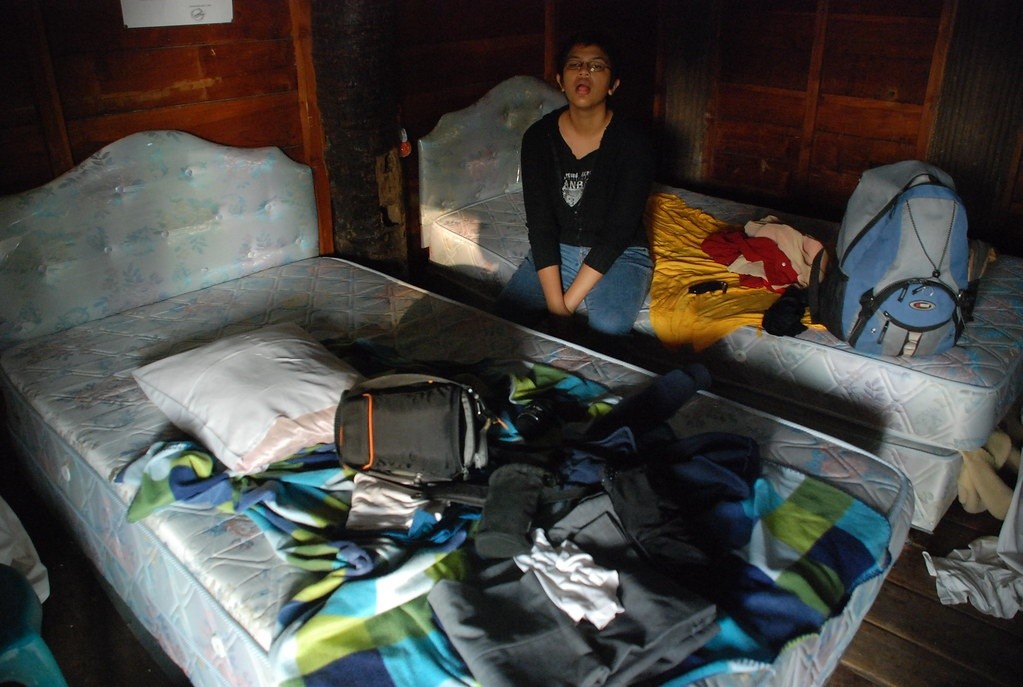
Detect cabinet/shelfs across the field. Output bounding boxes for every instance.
[702,0,960,222]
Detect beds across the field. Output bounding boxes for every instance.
[418,74,1023,538]
[0,130,915,686]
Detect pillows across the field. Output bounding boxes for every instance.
[130,320,370,480]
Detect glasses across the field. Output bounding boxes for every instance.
[561,60,613,72]
[688,280,728,294]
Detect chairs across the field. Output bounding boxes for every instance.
[0,564,69,687]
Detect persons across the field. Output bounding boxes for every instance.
[490,31,656,359]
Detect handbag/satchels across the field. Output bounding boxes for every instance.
[474,462,559,560]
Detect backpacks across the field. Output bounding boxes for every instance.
[811,158,970,358]
[333,371,501,508]
